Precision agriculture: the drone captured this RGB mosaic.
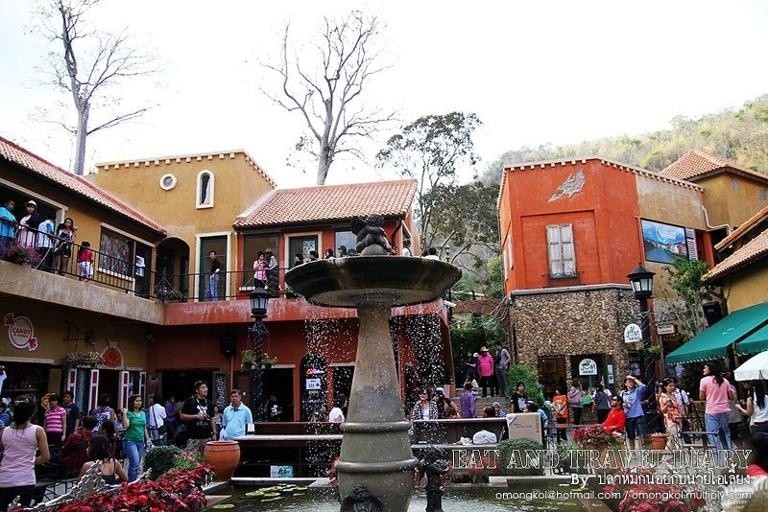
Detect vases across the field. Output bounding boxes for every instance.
[264,363,271,370]
[202,439,242,482]
[244,364,252,370]
[649,431,668,450]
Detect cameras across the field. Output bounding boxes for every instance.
[626,401,632,409]
[203,414,210,423]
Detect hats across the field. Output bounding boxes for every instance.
[480,346,490,353]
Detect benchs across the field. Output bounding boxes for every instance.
[7,459,153,512]
[36,435,127,503]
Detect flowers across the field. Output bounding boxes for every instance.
[154,286,175,299]
[239,349,257,365]
[64,350,100,368]
[573,424,626,452]
[1,237,42,266]
[260,352,280,364]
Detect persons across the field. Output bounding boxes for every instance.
[0,197,694,512]
[720,434,768,512]
[700,360,740,469]
[735,380,767,473]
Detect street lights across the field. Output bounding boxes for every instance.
[622,259,660,431]
[248,285,270,421]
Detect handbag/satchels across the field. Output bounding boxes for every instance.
[174,424,187,443]
[159,425,166,434]
